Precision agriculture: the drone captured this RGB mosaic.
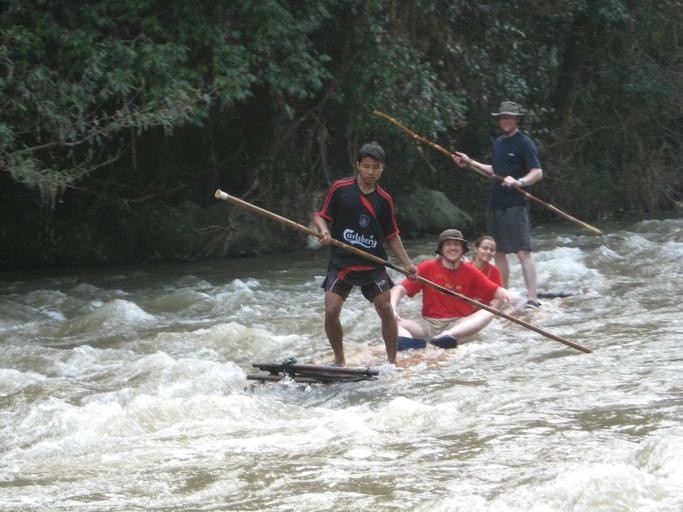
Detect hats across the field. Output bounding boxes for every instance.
[435,229,469,256]
[491,101,525,117]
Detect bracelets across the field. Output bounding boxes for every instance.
[519,177,528,186]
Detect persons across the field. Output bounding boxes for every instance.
[451,99,545,312]
[388,228,519,352]
[465,235,505,311]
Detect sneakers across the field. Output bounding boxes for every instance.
[524,301,542,310]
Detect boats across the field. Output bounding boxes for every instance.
[243,284,590,397]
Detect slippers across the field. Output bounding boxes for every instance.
[430,331,457,349]
[398,336,426,350]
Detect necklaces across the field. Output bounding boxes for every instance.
[311,140,418,368]
